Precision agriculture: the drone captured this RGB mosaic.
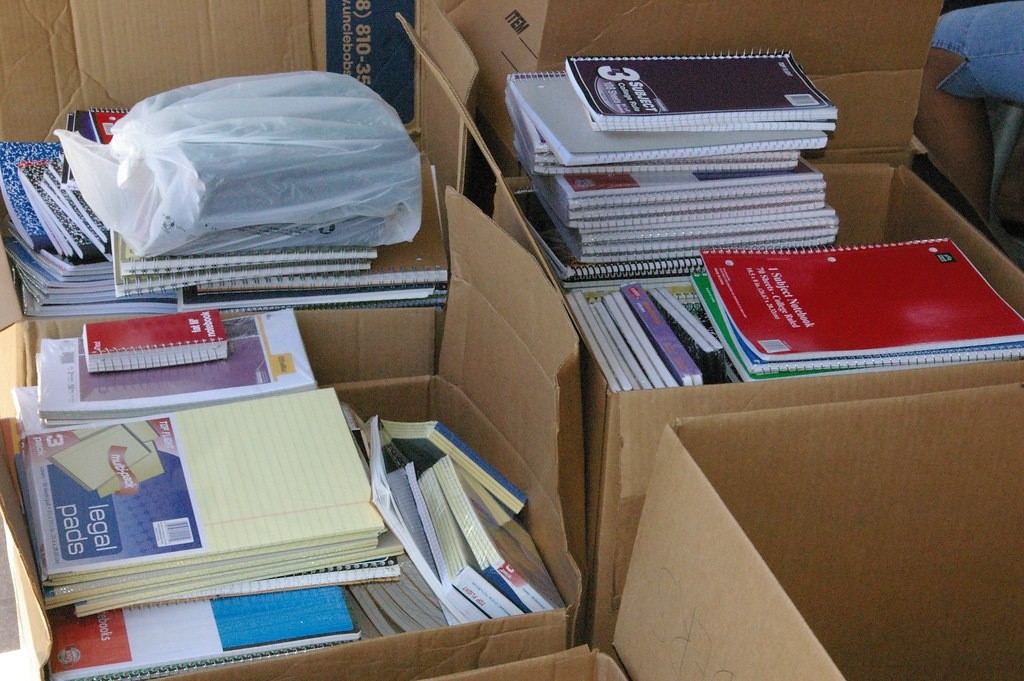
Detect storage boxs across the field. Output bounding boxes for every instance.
[0,0,1024,681]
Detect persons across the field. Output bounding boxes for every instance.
[913,1,1024,223]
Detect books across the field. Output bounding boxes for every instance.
[0,47,1024,681]
